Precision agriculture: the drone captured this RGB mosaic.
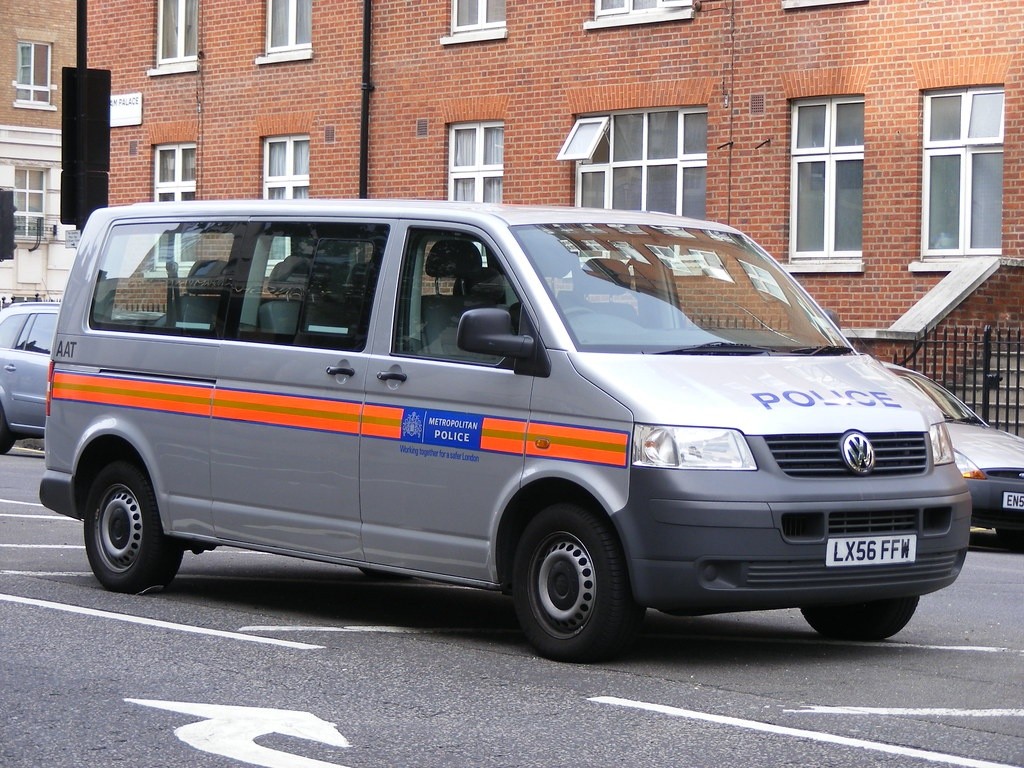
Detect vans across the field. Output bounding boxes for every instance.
[35,199,974,664]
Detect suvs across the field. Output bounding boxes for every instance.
[0,300,165,455]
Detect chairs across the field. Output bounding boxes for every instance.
[578,257,639,324]
[181,254,372,333]
[421,239,502,362]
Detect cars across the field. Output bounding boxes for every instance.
[878,360,1024,548]
